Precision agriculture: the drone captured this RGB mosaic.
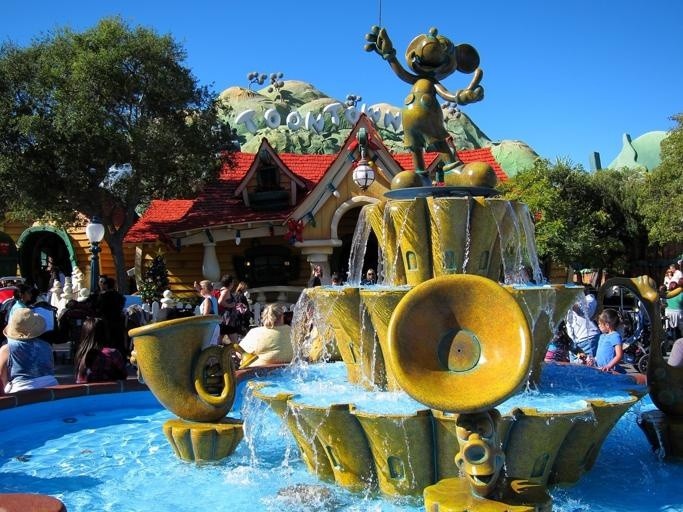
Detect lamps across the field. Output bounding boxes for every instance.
[235,230,241,245]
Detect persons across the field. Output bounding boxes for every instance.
[0,264,384,392]
[503,264,682,375]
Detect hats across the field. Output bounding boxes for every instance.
[3,308,46,340]
